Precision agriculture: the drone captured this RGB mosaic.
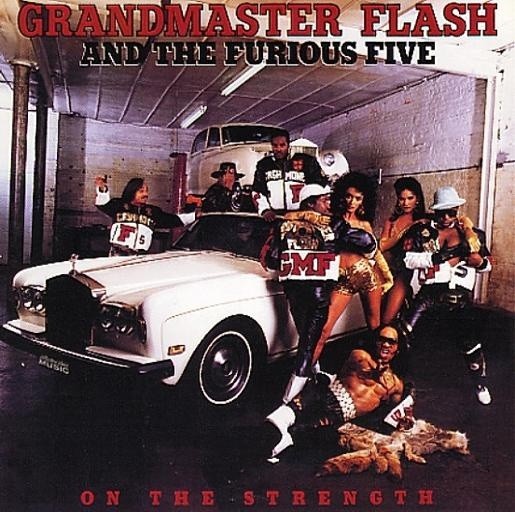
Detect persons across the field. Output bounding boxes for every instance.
[201,161,250,213]
[280,171,385,363]
[283,184,383,403]
[397,185,495,408]
[92,175,202,256]
[222,323,405,467]
[379,175,430,328]
[250,129,331,256]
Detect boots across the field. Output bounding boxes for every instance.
[282,373,308,404]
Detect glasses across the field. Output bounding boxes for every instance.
[435,210,457,217]
[375,336,399,345]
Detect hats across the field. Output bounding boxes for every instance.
[299,184,333,204]
[211,163,245,181]
[428,187,467,211]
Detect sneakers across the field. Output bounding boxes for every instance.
[475,385,492,404]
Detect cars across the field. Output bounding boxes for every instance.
[185,123,348,202]
[2,210,369,403]
